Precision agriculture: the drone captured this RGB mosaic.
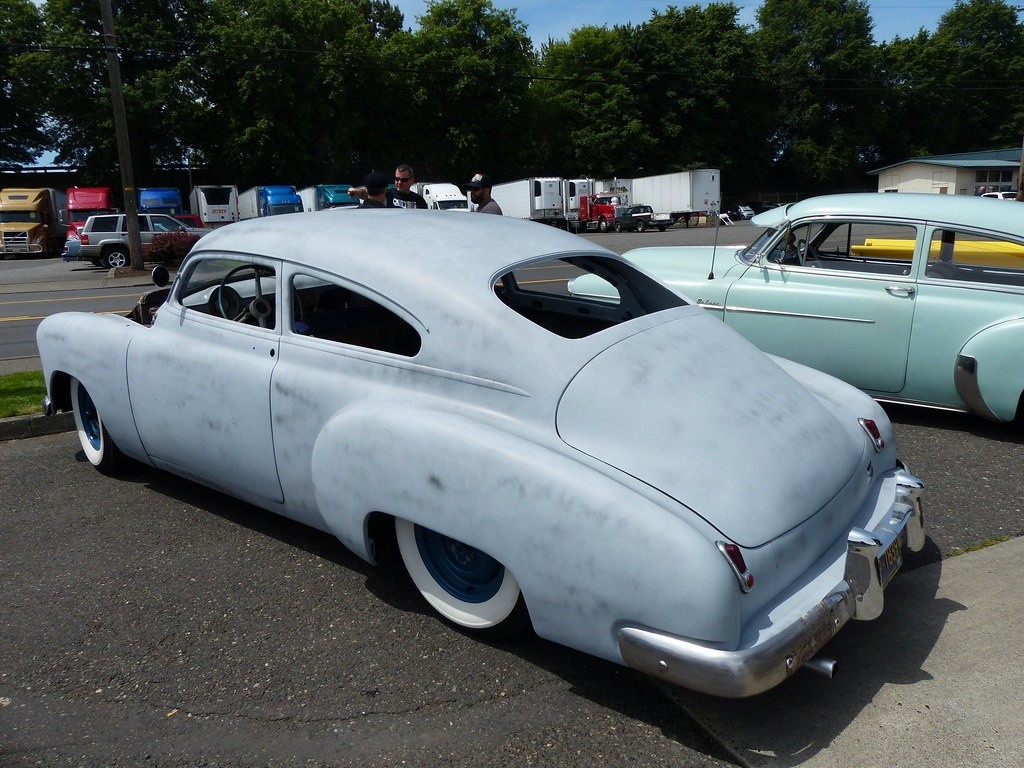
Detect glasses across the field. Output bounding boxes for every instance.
[393,176,413,183]
[467,187,487,191]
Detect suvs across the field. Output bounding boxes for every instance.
[78,210,215,271]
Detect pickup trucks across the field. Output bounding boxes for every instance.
[611,202,676,232]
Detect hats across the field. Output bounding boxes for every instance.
[465,174,492,188]
[364,172,389,189]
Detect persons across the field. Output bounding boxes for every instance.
[975,186,986,196]
[348,165,428,209]
[464,174,502,216]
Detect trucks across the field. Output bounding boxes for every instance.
[0,169,757,268]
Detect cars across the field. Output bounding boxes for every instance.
[35,207,927,700]
[566,192,1024,435]
[982,191,1018,202]
